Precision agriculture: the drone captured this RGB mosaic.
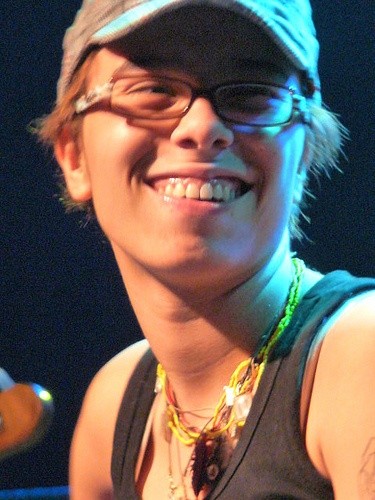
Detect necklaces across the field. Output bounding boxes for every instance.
[154,258,304,498]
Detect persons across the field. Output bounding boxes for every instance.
[24,0,375,499]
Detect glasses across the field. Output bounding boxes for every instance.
[75,75,307,127]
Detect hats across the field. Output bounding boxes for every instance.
[56,0,320,99]
